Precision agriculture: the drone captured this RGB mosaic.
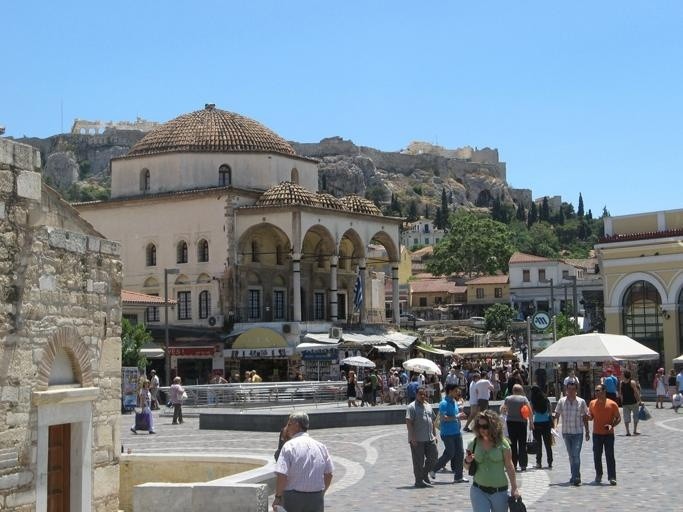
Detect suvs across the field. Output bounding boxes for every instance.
[399,313,423,322]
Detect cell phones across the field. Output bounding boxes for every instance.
[466,449,471,455]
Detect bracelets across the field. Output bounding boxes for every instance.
[275,496,281,498]
[464,459,472,464]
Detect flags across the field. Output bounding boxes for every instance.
[353,272,363,313]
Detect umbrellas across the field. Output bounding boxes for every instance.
[533,330,659,399]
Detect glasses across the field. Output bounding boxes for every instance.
[478,424,488,430]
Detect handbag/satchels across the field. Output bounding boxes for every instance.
[136,411,149,430]
[507,496,525,512]
[526,429,538,455]
[520,397,530,420]
[469,438,478,476]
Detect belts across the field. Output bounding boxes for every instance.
[473,481,508,495]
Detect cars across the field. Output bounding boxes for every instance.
[470,316,484,324]
[509,318,524,322]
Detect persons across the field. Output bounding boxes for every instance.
[339,343,683,436]
[530,385,555,469]
[272,411,333,512]
[434,389,464,473]
[274,424,291,462]
[429,384,469,483]
[583,384,621,485]
[404,386,439,488]
[463,409,527,512]
[131,369,263,434]
[499,384,535,471]
[553,382,590,486]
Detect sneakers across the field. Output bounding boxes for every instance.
[454,478,468,483]
[429,471,434,479]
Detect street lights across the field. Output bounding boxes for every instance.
[537,276,557,343]
[562,273,580,336]
[162,264,179,385]
